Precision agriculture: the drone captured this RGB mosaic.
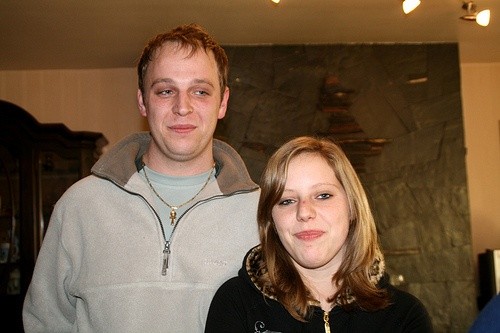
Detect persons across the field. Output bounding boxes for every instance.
[204,136,434,333]
[23,23,265,333]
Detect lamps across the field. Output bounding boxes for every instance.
[402,0,421,15]
[458,1,491,27]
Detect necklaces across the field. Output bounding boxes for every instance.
[141,162,215,226]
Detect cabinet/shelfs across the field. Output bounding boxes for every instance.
[0,98,109,333]
